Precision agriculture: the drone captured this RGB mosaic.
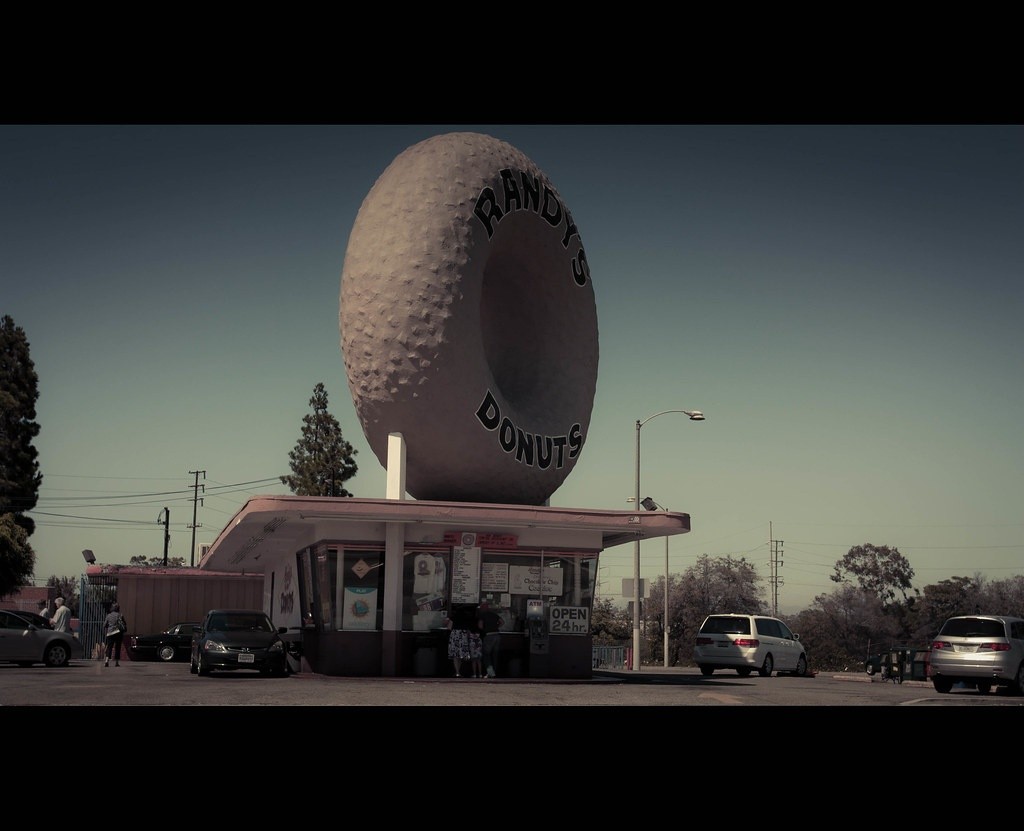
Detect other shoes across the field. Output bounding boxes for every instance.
[479,674,483,678]
[116,664,121,667]
[456,674,461,677]
[105,662,109,667]
[471,675,477,678]
[484,674,496,679]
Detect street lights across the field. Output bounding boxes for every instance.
[626,497,670,670]
[632,409,705,671]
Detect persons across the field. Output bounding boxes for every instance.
[38,599,49,626]
[50,597,70,634]
[448,604,483,677]
[102,603,126,667]
[479,603,505,678]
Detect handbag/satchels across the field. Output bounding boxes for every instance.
[114,613,127,632]
[107,628,120,640]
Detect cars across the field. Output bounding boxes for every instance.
[4,609,74,635]
[130,621,204,662]
[190,606,288,678]
[0,609,85,668]
[928,614,1024,697]
[865,651,889,676]
[694,613,809,678]
